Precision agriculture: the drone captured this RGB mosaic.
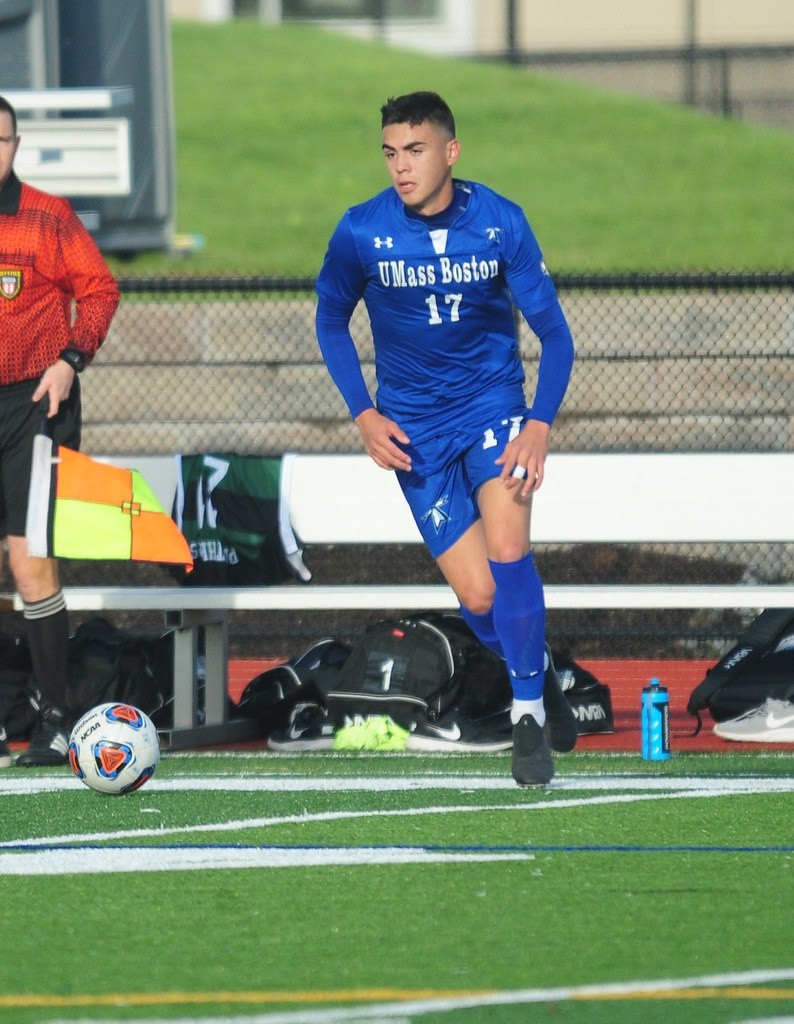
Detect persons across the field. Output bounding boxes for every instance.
[0,96,121,768]
[315,92,580,788]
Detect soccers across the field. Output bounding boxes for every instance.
[68,702,160,796]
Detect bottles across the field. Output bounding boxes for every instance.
[640,678,670,763]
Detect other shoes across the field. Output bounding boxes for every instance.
[541,639,578,755]
[508,704,554,787]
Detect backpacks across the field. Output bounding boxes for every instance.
[0,617,236,730]
[686,607,794,737]
[154,609,617,755]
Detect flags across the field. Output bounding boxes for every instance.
[26,433,195,576]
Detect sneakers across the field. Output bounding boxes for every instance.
[713,697,794,742]
[13,703,79,767]
